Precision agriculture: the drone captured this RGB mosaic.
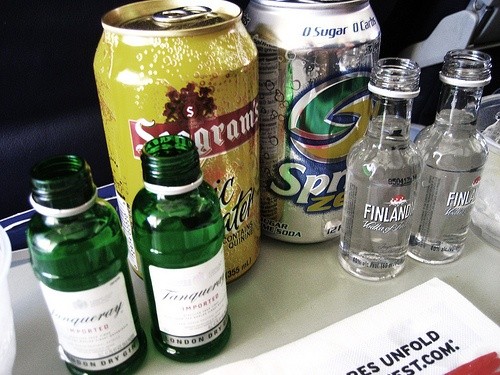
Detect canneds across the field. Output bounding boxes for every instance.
[92,0,380,287]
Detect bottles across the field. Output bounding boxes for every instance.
[131,135,232,363]
[26,156,148,375]
[406,48,492,264]
[337,57,422,280]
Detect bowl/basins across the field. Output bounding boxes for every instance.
[468,93,500,249]
